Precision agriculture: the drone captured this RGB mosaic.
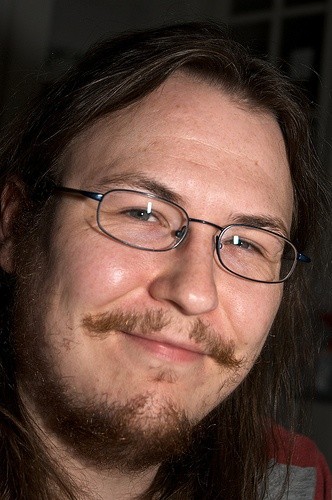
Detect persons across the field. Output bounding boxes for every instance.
[0,17,332,499]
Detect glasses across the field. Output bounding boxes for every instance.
[48,183,312,284]
